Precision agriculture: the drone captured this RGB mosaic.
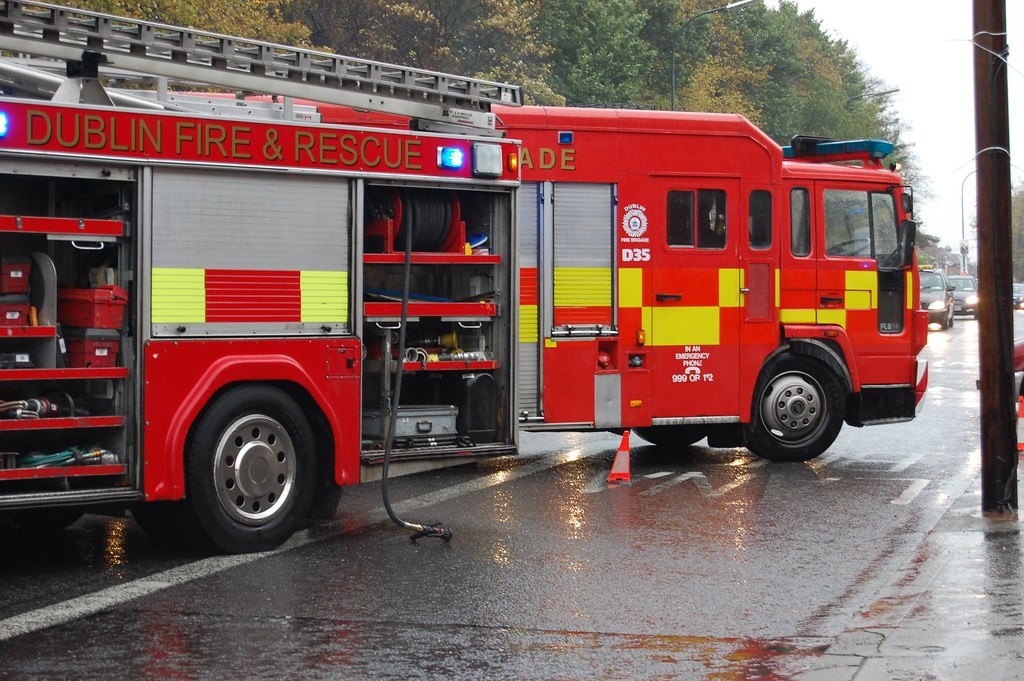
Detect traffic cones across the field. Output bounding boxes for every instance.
[1012,394,1024,451]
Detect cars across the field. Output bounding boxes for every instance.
[1011,281,1024,310]
[915,266,954,329]
[942,273,977,321]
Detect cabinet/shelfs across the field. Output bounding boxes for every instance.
[0,210,504,482]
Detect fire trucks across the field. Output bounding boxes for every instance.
[0,2,928,555]
